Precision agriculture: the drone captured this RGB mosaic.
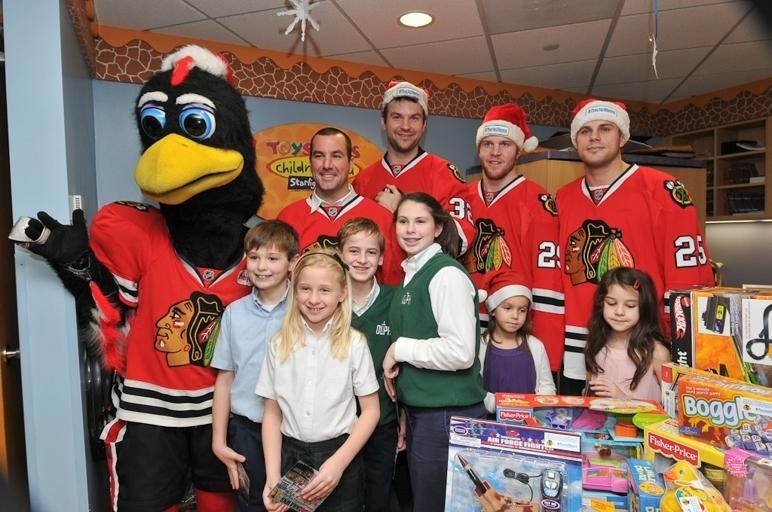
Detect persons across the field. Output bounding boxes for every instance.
[154,290,227,367]
[254,246,381,512]
[352,80,477,259]
[335,216,407,512]
[275,127,407,287]
[210,219,300,512]
[382,192,488,512]
[554,98,715,396]
[478,266,557,421]
[564,215,635,287]
[444,102,565,396]
[582,267,672,404]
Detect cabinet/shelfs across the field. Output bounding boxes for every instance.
[667,115,772,221]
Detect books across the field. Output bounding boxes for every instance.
[736,141,766,151]
[722,161,766,217]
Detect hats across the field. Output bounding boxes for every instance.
[486,268,533,312]
[382,78,433,116]
[570,98,631,149]
[476,103,539,154]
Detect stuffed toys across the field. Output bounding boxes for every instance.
[7,44,264,512]
